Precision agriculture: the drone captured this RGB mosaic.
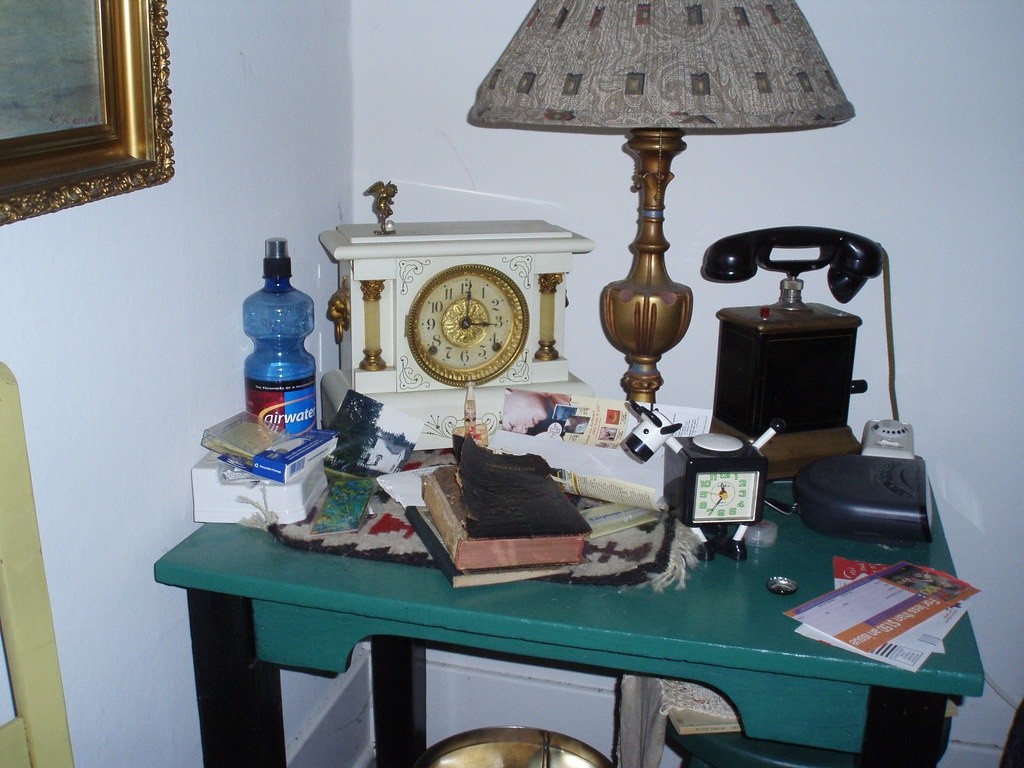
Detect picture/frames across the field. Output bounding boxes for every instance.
[0,0,176,226]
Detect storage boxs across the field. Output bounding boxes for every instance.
[190,450,329,524]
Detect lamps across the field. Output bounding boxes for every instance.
[467,0,855,402]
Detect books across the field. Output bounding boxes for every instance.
[404,505,573,589]
[424,433,593,568]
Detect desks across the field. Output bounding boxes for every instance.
[155,485,985,768]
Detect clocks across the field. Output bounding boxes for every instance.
[626,398,787,561]
[318,220,595,443]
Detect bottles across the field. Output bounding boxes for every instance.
[242,238,317,436]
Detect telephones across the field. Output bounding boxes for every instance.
[701,225,885,484]
